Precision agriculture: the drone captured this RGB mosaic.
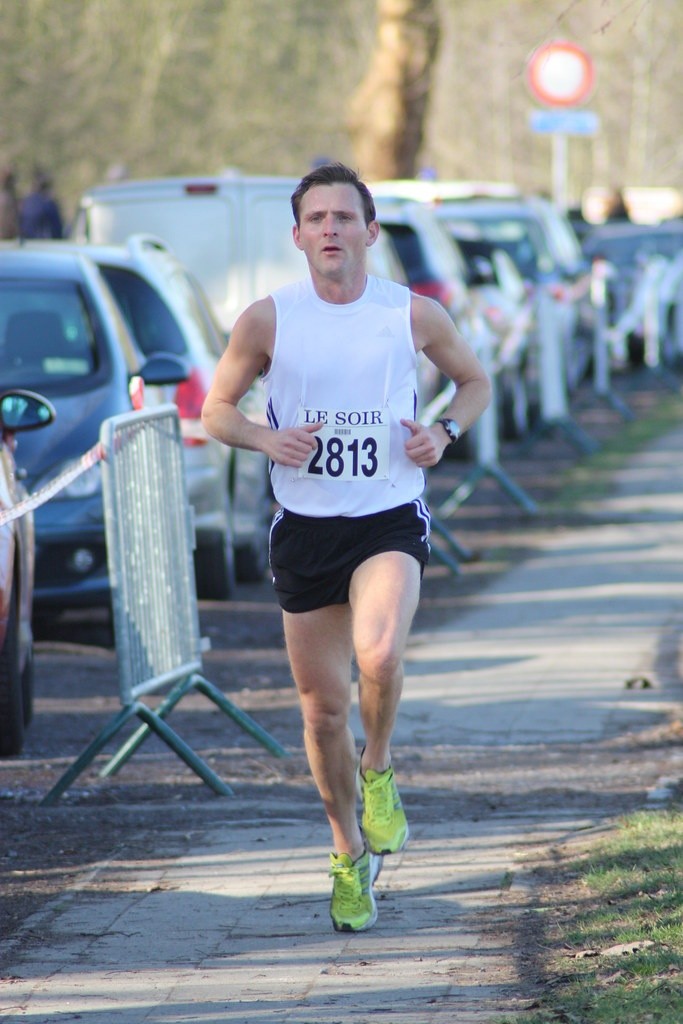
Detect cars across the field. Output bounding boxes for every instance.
[1,246,193,636]
[368,178,683,471]
[0,385,58,758]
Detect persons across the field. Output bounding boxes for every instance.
[201,164,492,932]
[0,167,64,238]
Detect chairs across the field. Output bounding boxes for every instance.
[4,309,68,371]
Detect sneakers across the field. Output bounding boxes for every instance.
[326,850,384,932]
[355,744,410,857]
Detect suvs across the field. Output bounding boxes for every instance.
[33,233,273,600]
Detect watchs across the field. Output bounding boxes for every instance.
[434,417,461,444]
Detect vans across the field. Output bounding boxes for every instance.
[69,172,412,395]
